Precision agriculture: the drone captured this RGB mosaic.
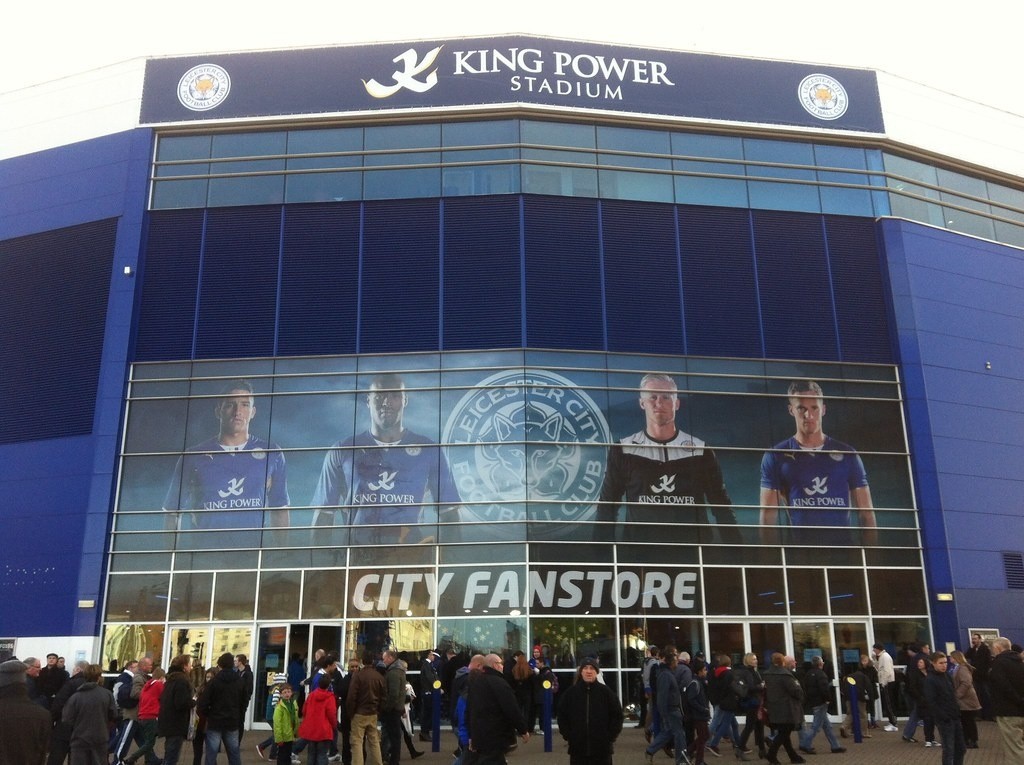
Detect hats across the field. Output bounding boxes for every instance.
[873,643,883,650]
[0,660,31,673]
[534,646,542,657]
[579,657,600,675]
[696,651,705,657]
[678,651,690,661]
[217,652,235,670]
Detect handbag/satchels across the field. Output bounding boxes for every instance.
[187,707,200,741]
[757,707,768,721]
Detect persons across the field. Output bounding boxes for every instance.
[309,372,464,553]
[557,653,624,765]
[840,642,898,738]
[0,652,253,765]
[759,380,879,545]
[902,643,982,765]
[559,649,575,696]
[964,634,1024,765]
[161,381,290,577]
[593,373,741,559]
[419,645,558,765]
[257,648,426,765]
[633,645,847,765]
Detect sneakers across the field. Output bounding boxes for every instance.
[882,722,899,732]
[256,743,264,759]
[924,741,942,747]
[112,760,129,765]
[268,756,278,761]
[682,750,694,765]
[902,735,918,743]
[704,745,723,757]
[733,743,753,754]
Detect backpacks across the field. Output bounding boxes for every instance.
[545,669,559,693]
[117,682,139,710]
[679,679,700,721]
[706,667,731,706]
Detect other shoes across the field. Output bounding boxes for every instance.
[328,754,342,763]
[145,758,165,765]
[966,741,979,748]
[452,748,462,759]
[411,751,425,759]
[382,756,391,765]
[758,736,847,764]
[419,733,433,742]
[535,730,544,736]
[840,725,849,739]
[634,722,674,765]
[291,752,300,759]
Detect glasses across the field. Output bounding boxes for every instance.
[495,661,504,665]
